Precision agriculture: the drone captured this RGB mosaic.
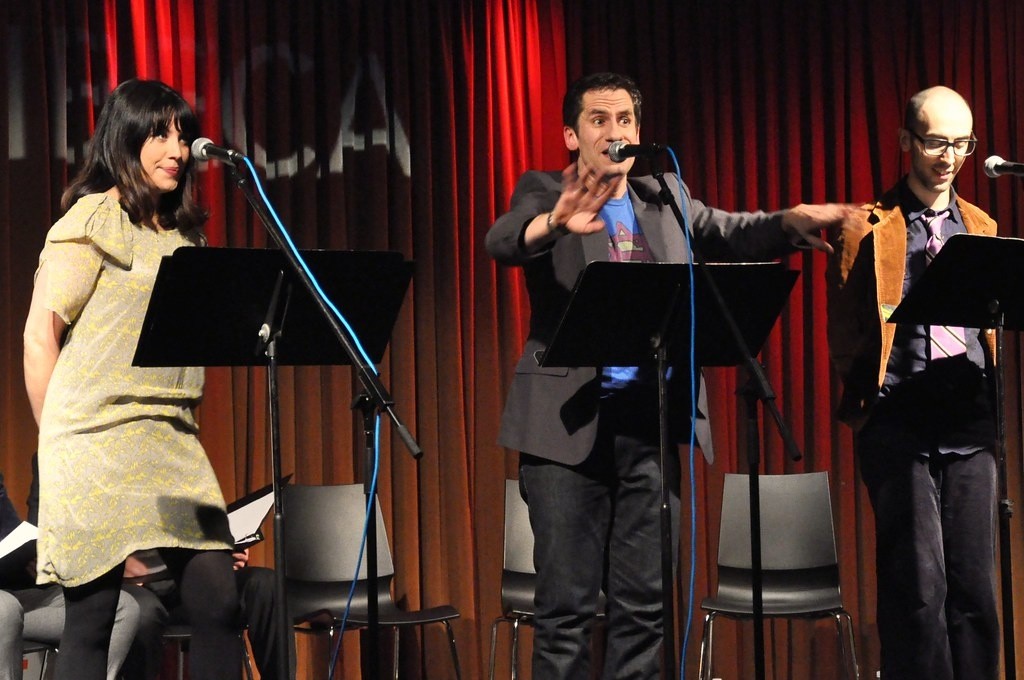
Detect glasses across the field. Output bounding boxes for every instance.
[903,124,977,157]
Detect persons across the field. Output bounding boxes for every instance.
[485,72,851,679]
[0,471,139,680]
[24,80,243,680]
[28,456,297,680]
[828,87,1000,679]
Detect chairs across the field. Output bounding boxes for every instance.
[22,623,255,680]
[697,471,860,680]
[489,479,607,680]
[282,483,462,680]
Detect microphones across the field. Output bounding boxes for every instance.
[190,137,248,162]
[983,156,1024,178]
[609,140,670,163]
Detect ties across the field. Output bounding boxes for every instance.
[919,210,967,368]
